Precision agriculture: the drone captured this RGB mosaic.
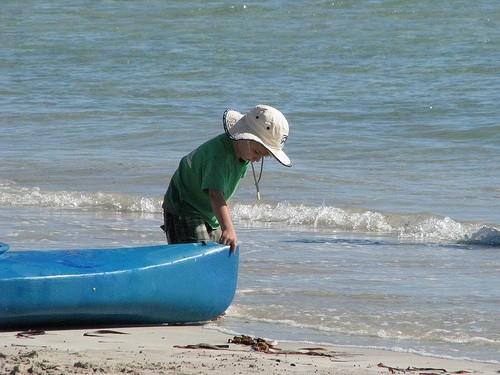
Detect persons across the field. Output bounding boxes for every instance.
[160,105,292,321]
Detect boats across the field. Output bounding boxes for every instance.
[0,239,239,324]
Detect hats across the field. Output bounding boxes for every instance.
[223,104,293,167]
